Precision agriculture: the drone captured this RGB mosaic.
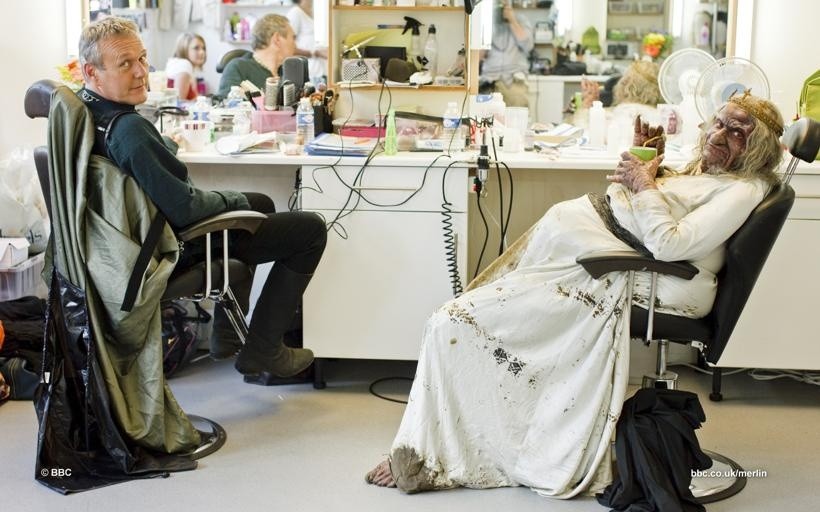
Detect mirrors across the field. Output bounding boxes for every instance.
[479,0,737,127]
[81,1,330,105]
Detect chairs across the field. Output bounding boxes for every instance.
[23,79,272,460]
[575,117,820,505]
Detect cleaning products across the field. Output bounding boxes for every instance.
[425,23,438,84]
[587,97,607,147]
[402,15,425,71]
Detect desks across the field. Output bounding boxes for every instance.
[528,74,621,126]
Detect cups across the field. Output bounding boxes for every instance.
[502,107,529,152]
[182,120,209,151]
[630,147,656,160]
[278,131,302,155]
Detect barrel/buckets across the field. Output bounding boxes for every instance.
[504,106,531,154]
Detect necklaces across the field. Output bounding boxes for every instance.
[492,26,511,51]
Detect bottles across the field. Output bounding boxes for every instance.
[442,103,461,158]
[588,100,605,150]
[583,50,591,63]
[223,11,257,42]
[458,50,466,70]
[227,85,242,109]
[197,77,205,96]
[193,96,211,147]
[657,103,681,135]
[570,51,577,62]
[296,97,316,153]
[524,130,535,151]
[700,22,710,43]
[421,24,438,80]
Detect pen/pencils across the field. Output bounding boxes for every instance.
[353,139,372,145]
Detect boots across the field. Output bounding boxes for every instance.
[208,261,258,362]
[232,257,314,379]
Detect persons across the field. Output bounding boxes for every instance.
[75,17,328,378]
[366,93,784,488]
[458,0,534,81]
[219,13,297,99]
[568,61,664,150]
[165,31,207,99]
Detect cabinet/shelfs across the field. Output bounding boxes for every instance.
[216,1,296,45]
[171,122,819,403]
[328,5,478,94]
